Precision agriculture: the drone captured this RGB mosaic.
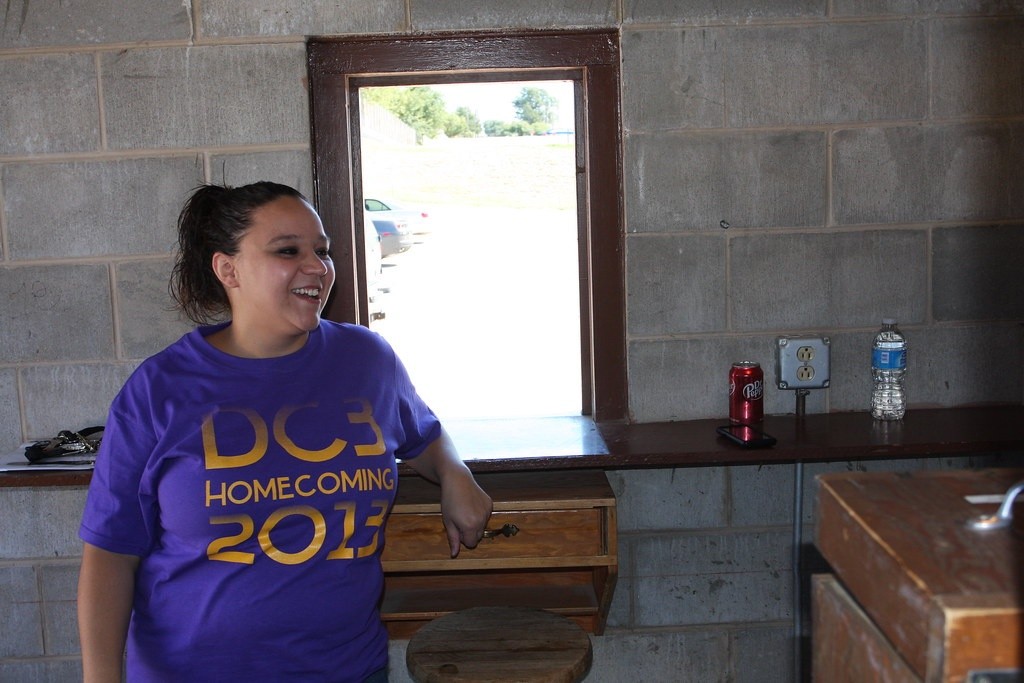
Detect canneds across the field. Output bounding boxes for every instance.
[729,360,764,424]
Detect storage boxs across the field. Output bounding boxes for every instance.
[812,469,1024,683]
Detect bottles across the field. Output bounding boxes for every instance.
[871,318,908,420]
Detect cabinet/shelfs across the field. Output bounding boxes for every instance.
[374,469,620,641]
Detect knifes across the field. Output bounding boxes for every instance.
[7,460,97,465]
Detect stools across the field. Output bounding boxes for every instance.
[408,606,595,683]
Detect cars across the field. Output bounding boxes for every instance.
[364,194,433,260]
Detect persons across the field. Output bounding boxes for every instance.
[78,180,493,683]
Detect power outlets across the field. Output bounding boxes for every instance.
[775,333,831,390]
